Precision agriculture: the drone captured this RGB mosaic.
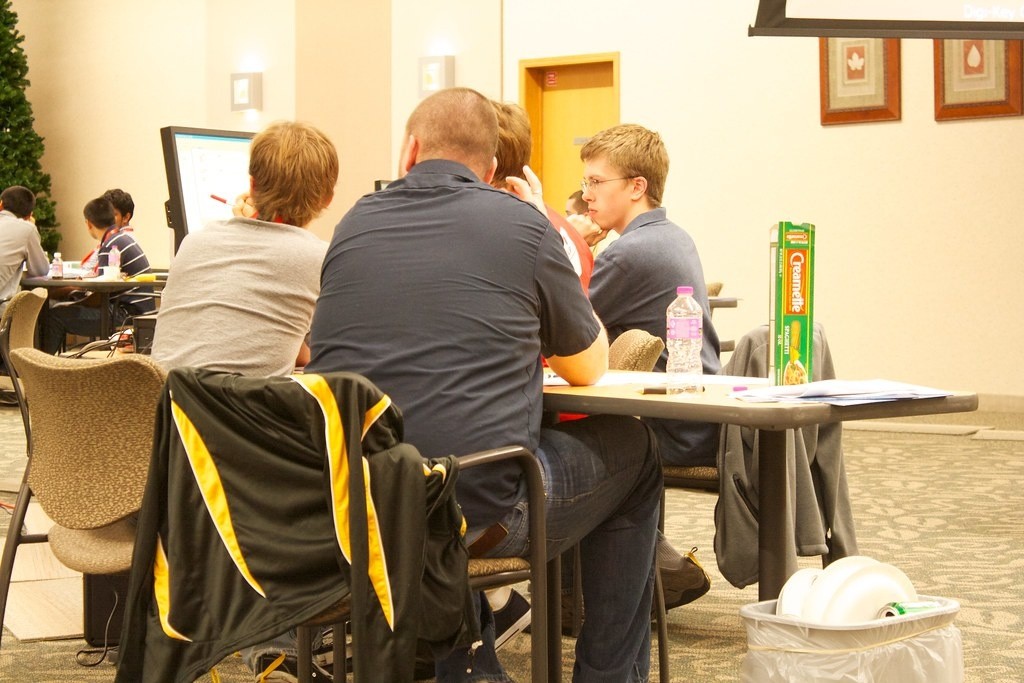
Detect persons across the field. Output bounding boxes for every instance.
[510,126,725,633]
[304,88,665,683]
[153,123,339,683]
[481,99,622,656]
[0,185,156,373]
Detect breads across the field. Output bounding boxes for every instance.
[789,321,800,363]
[608,329,665,373]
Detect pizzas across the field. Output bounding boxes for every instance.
[785,363,806,385]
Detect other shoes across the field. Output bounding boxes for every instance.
[493,590,530,653]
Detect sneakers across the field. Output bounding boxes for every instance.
[560,592,586,636]
[650,546,710,621]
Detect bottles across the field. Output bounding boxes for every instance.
[52,252,63,280]
[665,286,704,399]
[109,245,120,267]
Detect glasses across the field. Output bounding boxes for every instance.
[580,173,640,195]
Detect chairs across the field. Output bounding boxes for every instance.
[0,264,860,683]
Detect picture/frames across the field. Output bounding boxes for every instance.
[934,39,1024,122]
[820,36,902,126]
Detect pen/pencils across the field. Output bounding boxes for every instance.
[644,386,706,394]
[209,194,236,208]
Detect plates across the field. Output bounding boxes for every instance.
[775,556,918,626]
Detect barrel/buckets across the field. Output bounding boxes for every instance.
[740,596,961,682]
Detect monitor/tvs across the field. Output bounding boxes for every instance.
[160,127,259,257]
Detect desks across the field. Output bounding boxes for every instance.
[21,273,167,350]
[541,373,977,601]
